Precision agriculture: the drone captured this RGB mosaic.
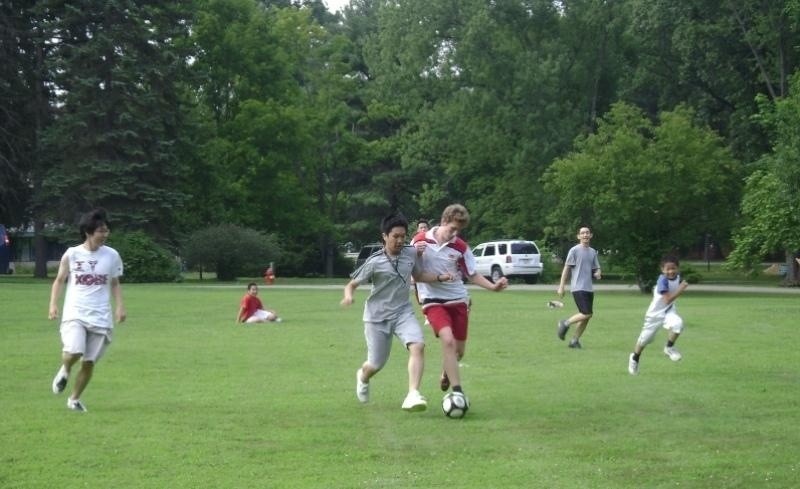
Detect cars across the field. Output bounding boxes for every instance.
[337,242,362,263]
[0,223,11,276]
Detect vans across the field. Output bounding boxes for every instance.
[356,245,416,271]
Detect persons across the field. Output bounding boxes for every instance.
[48,208,126,412]
[408,203,508,394]
[411,221,432,326]
[237,283,282,323]
[556,223,602,349]
[340,213,456,415]
[627,255,689,375]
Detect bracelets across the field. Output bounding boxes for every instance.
[437,274,443,282]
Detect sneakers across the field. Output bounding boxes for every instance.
[357,370,369,402]
[402,397,428,412]
[569,338,581,348]
[629,353,639,374]
[664,345,681,361]
[558,320,568,340]
[68,398,86,413]
[441,370,449,391]
[52,366,69,394]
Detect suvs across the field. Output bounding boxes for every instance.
[471,240,544,285]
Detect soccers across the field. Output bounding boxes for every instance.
[442,392,467,419]
